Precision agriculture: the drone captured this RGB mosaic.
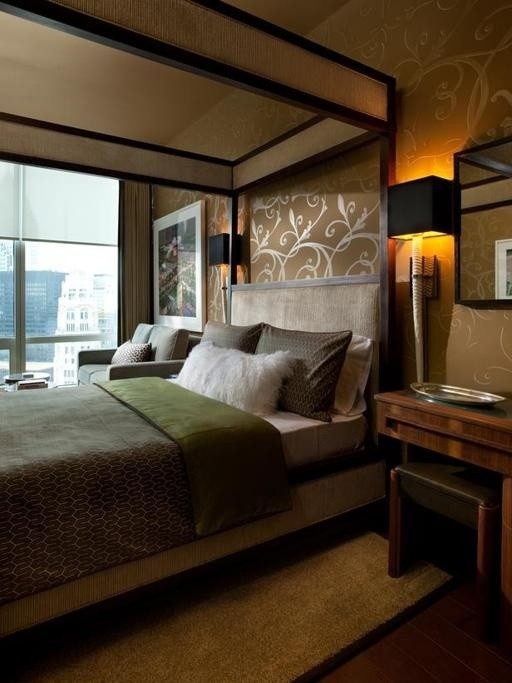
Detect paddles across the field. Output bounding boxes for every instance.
[28,529,454,683]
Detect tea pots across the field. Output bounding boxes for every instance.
[452,135,511,307]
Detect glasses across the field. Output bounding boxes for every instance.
[387,176,457,386]
[208,233,243,323]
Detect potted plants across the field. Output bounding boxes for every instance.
[149,198,208,335]
[494,239,511,299]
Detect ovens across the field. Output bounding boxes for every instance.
[0,366,397,649]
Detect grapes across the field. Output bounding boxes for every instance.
[374,389,512,619]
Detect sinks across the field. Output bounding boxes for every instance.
[78,323,190,384]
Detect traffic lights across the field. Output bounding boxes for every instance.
[111,338,152,366]
[252,325,353,423]
[177,341,297,416]
[199,320,263,352]
[333,333,374,418]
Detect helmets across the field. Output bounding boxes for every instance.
[410,383,507,406]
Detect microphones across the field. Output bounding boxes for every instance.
[389,461,499,642]
